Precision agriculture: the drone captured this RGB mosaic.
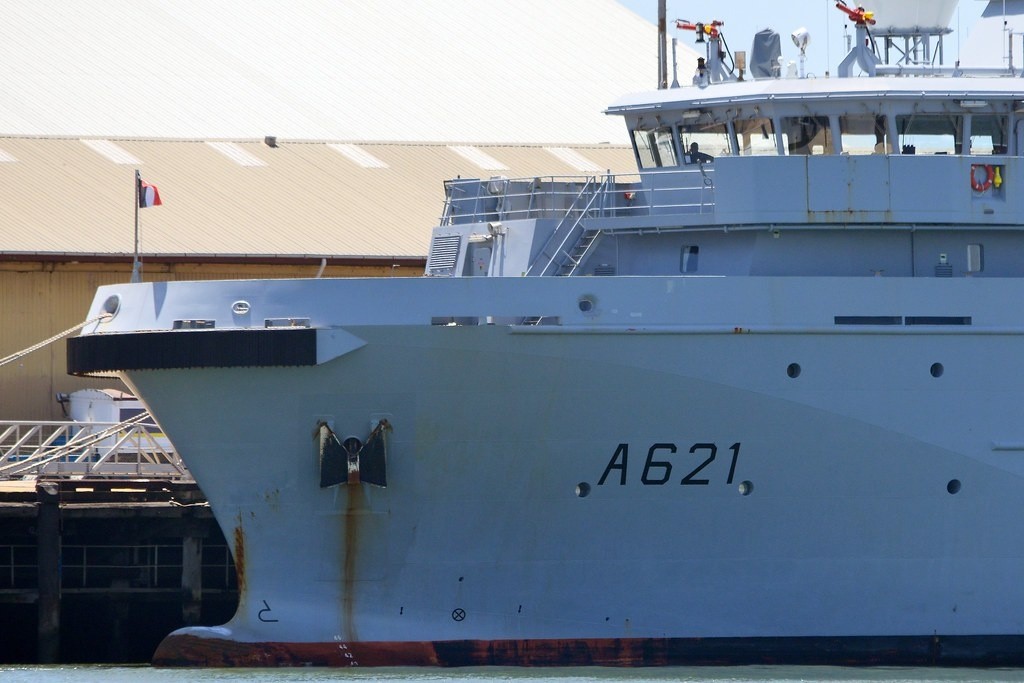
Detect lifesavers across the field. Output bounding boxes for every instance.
[971,164,994,192]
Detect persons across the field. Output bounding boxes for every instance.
[685,142,713,163]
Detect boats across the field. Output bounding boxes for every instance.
[65,0,1024,668]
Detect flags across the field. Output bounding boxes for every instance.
[139,179,162,208]
